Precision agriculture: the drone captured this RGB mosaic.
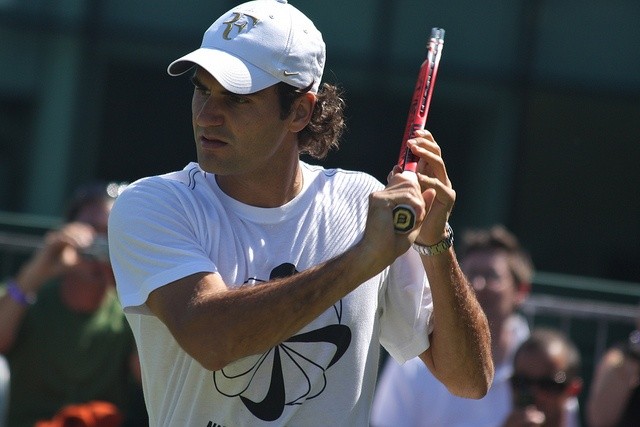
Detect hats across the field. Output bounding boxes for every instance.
[166,0,327,98]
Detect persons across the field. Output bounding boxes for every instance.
[585,330,640,426]
[0,191,150,426]
[504,327,584,427]
[369,224,532,426]
[107,0,495,426]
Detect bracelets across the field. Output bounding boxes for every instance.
[6,278,36,308]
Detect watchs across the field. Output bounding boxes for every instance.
[412,224,455,256]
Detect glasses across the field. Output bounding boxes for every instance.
[509,372,577,393]
